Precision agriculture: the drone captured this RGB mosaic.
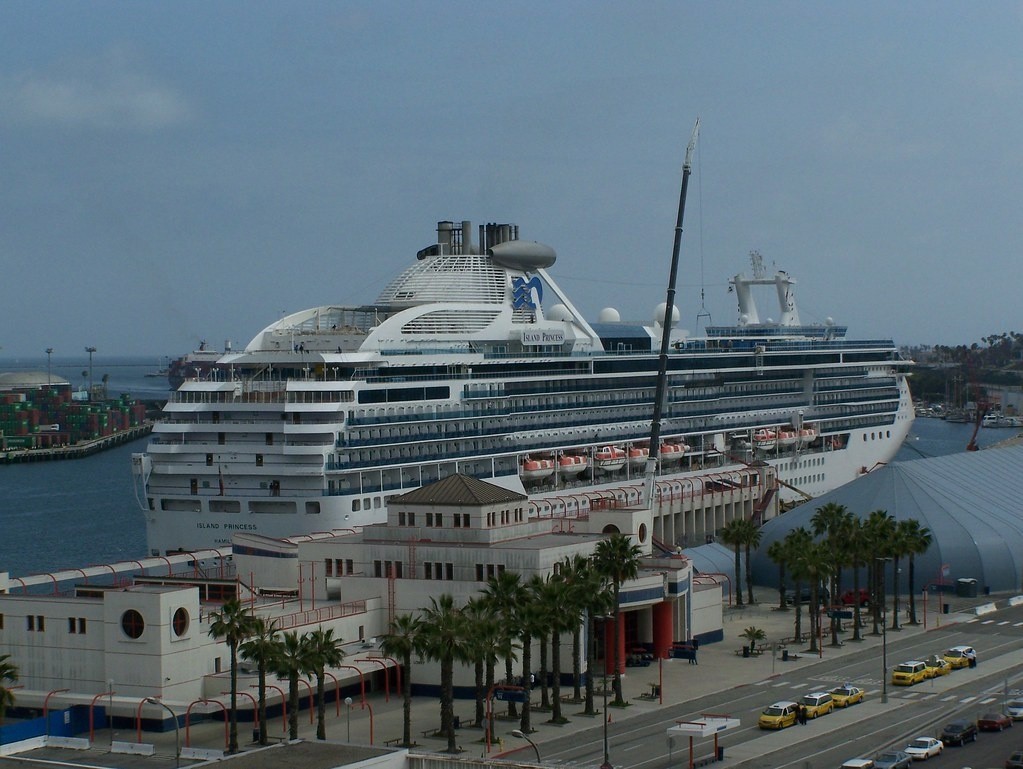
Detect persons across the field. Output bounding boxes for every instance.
[295,344,298,353]
[967,648,976,669]
[299,345,303,351]
[338,347,342,353]
[905,602,910,617]
[795,703,808,725]
[332,324,336,328]
[688,646,697,665]
[530,673,535,690]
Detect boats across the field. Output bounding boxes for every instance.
[522,439,691,480]
[915,402,1023,428]
[754,427,819,451]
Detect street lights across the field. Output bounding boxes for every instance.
[146,696,180,769]
[107,679,114,747]
[875,558,893,704]
[595,615,615,763]
[45,348,54,389]
[344,697,352,742]
[83,346,96,392]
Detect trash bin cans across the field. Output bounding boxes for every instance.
[743,646,749,658]
[985,585,990,595]
[943,604,950,614]
[782,650,788,661]
[453,716,459,729]
[253,728,259,741]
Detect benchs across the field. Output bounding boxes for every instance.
[560,693,572,699]
[110,740,157,757]
[266,736,285,743]
[47,735,92,750]
[178,747,224,761]
[459,719,474,727]
[734,617,867,656]
[529,701,540,708]
[494,709,507,718]
[420,728,439,736]
[383,737,402,747]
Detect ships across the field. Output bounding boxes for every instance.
[132,211,919,597]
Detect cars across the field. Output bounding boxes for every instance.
[943,646,976,668]
[784,587,873,607]
[799,692,834,719]
[830,683,865,708]
[840,736,944,769]
[759,701,800,730]
[925,655,951,678]
[940,720,977,747]
[893,661,926,685]
[977,698,1023,731]
[1005,750,1023,769]
